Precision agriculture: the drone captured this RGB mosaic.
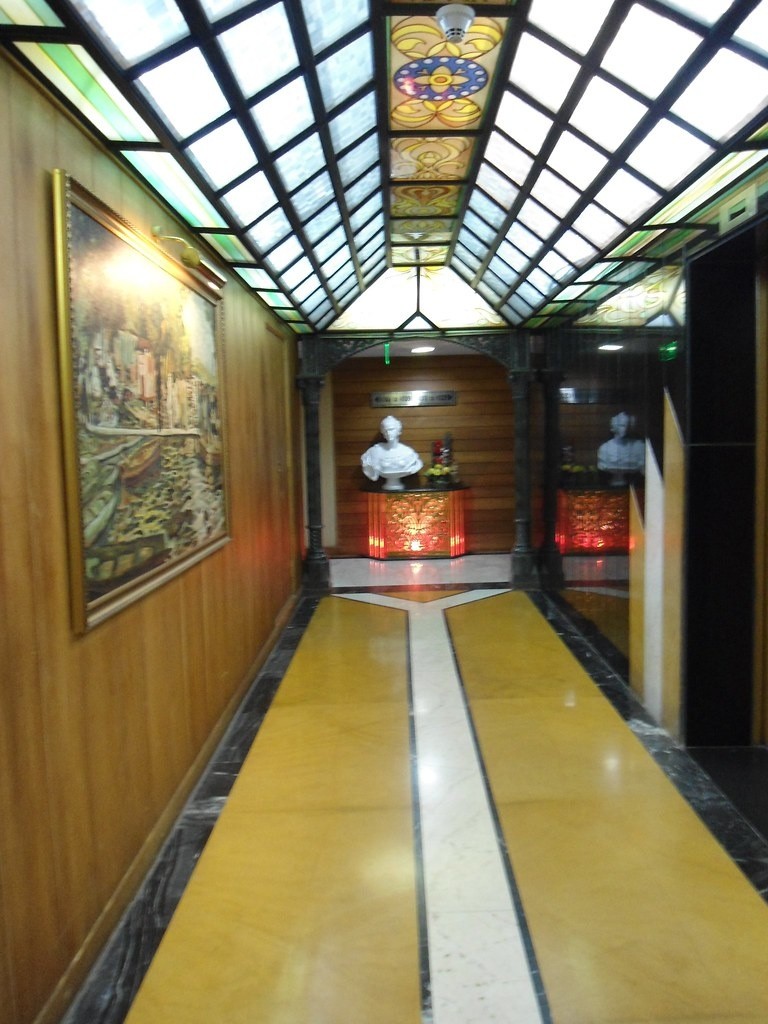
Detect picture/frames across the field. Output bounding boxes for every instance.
[51,165,231,634]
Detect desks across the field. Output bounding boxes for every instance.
[362,484,470,560]
[554,471,647,558]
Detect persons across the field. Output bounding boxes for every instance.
[596,411,646,471]
[359,415,424,482]
